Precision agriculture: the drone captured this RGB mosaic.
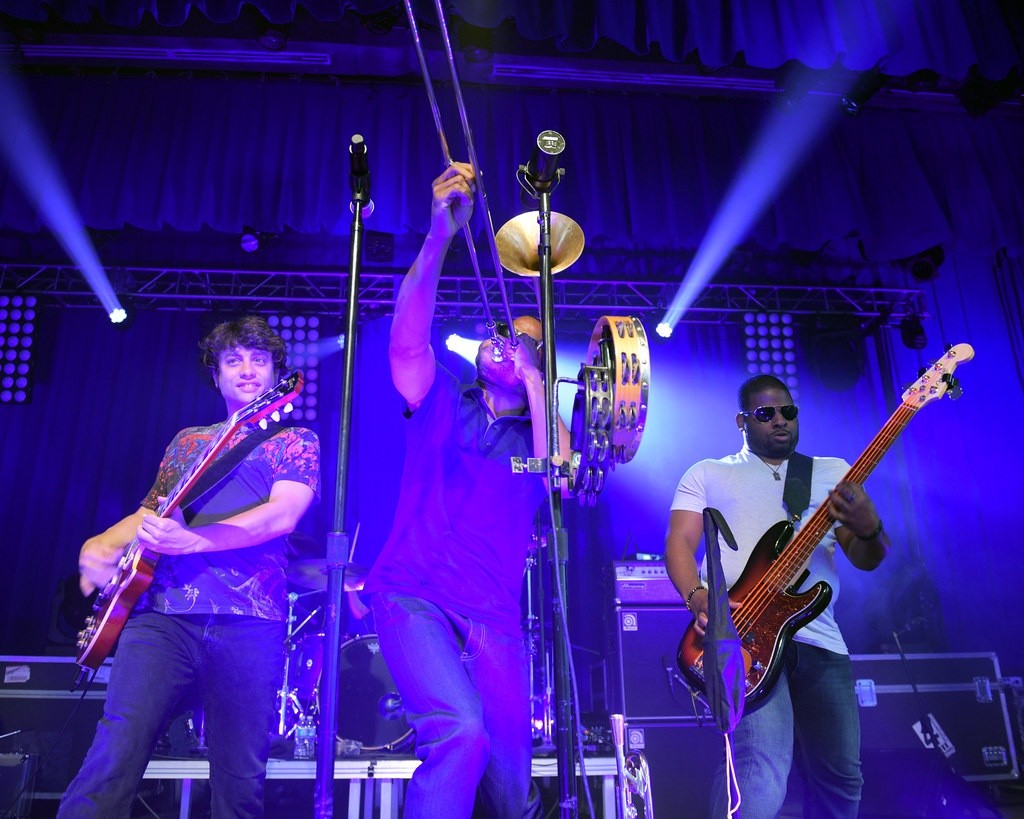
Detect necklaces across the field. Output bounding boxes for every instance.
[754,452,786,480]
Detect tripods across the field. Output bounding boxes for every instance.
[276,593,304,735]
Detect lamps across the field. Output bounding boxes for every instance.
[110,307,134,332]
[651,322,673,346]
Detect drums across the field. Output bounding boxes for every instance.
[288,629,325,702]
[313,634,414,752]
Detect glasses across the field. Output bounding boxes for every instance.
[741,405,800,423]
[496,321,543,349]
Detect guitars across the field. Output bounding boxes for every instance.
[75,371,307,670]
[676,342,975,718]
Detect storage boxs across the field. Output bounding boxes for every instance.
[846,652,1018,784]
[0,650,194,798]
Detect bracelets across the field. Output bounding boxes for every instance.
[686,586,705,611]
[856,520,883,540]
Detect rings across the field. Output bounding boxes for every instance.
[518,331,522,335]
[849,494,856,502]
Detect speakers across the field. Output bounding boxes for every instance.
[0,694,107,799]
[607,605,714,721]
[623,723,725,819]
[0,752,39,819]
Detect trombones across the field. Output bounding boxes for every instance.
[404,0,584,363]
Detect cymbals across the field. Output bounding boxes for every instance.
[284,560,370,592]
[568,315,650,507]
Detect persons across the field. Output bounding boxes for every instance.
[665,375,890,818]
[362,162,581,819]
[54,318,322,819]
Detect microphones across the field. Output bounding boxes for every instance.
[349,134,374,218]
[520,130,565,208]
[384,698,402,713]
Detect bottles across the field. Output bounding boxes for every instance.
[305,715,316,759]
[293,715,308,760]
